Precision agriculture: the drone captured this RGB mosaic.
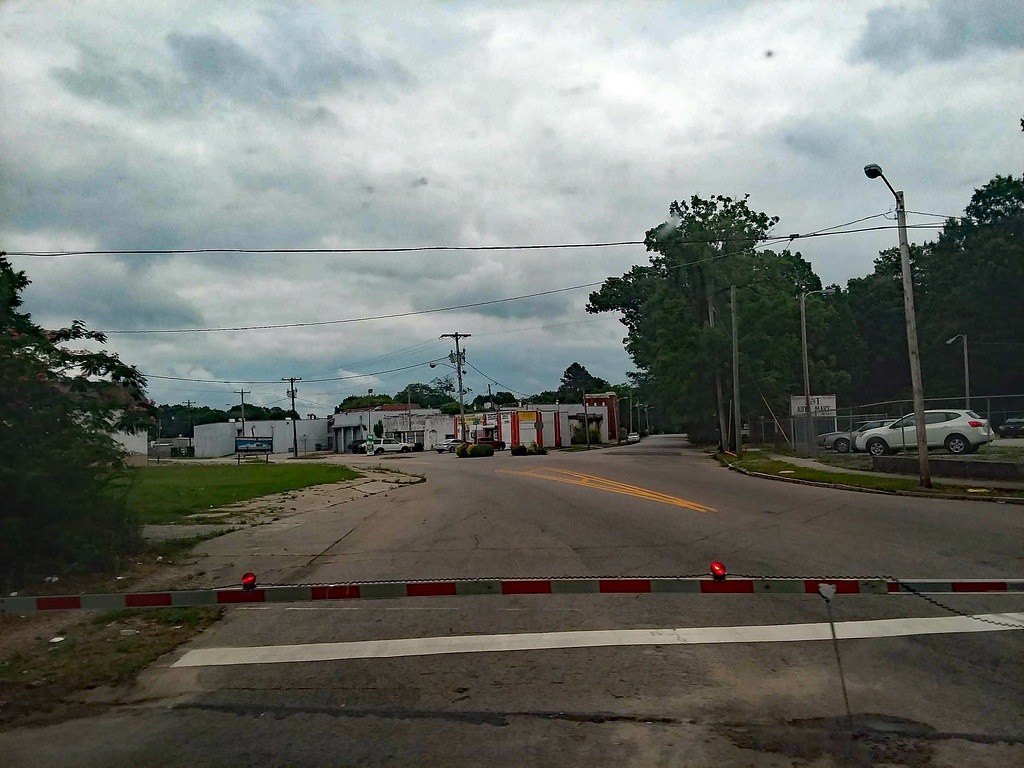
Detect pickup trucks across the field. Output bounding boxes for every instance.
[627,432,640,444]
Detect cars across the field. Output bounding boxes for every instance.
[997,413,1024,438]
[855,409,996,457]
[434,439,463,454]
[467,437,506,452]
[815,419,900,453]
[351,439,370,454]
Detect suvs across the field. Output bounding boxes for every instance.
[365,438,410,455]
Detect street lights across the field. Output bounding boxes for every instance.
[801,289,836,417]
[368,388,373,434]
[613,397,654,444]
[864,162,932,487]
[430,361,466,444]
[945,333,971,411]
